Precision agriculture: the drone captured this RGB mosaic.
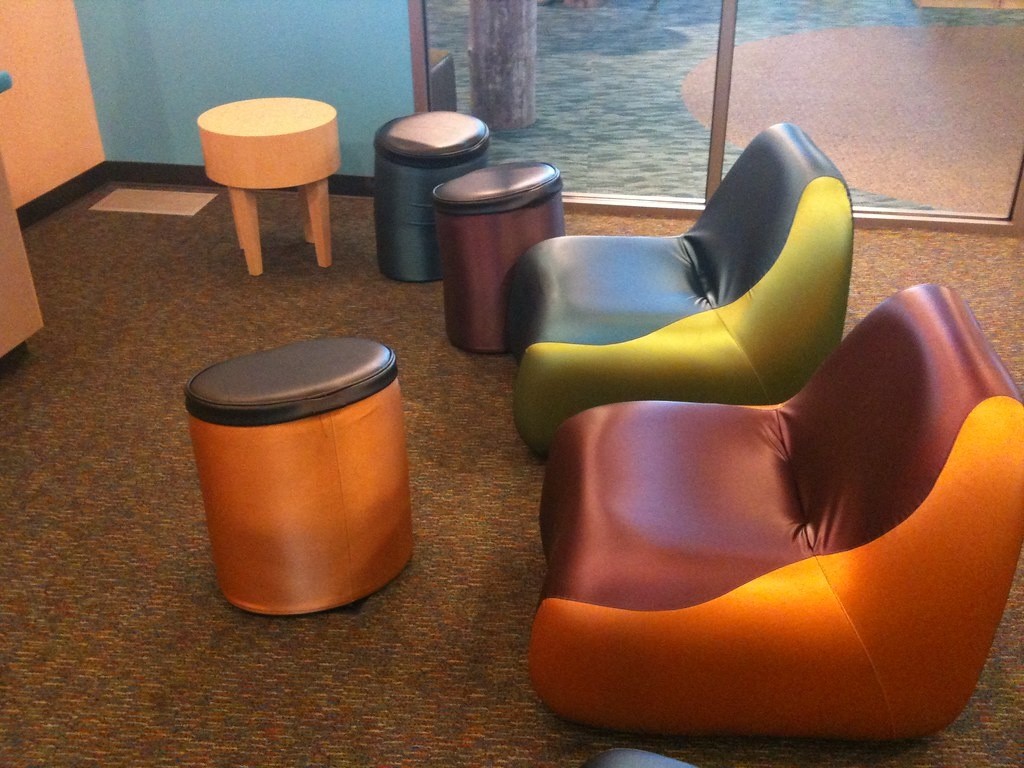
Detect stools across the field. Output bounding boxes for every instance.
[374,108,490,284]
[432,158,566,355]
[183,336,414,616]
[197,96,340,277]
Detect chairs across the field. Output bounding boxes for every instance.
[504,122,855,459]
[528,282,1024,744]
